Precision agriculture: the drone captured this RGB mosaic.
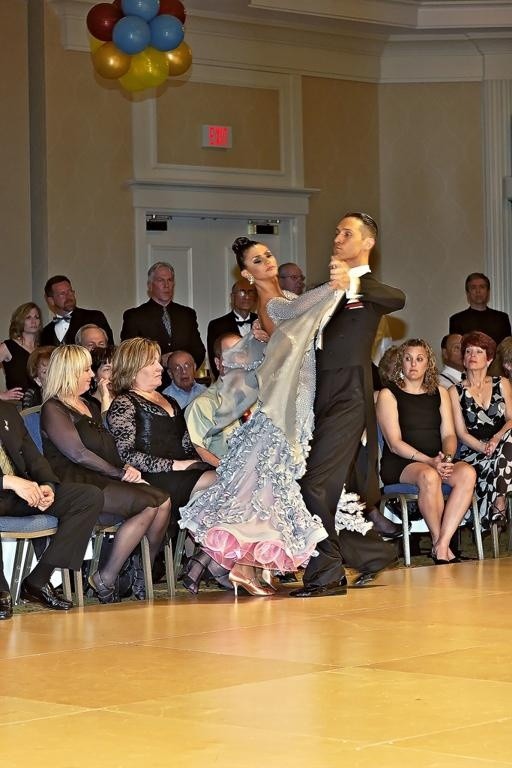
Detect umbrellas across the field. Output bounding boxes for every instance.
[20,579,73,613]
[351,557,401,587]
[377,522,413,539]
[287,573,347,599]
[1,595,13,619]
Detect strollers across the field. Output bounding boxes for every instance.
[239,317,257,326]
[52,314,73,325]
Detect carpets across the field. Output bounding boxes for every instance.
[161,307,173,338]
[461,373,468,380]
[0,444,15,477]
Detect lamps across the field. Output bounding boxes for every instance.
[375,400,484,565]
[490,493,512,560]
[101,410,185,594]
[1,515,70,610]
[20,404,153,606]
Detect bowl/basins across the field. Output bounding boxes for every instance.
[133,388,160,403]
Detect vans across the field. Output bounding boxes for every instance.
[83,569,121,602]
[121,554,146,604]
[229,569,281,600]
[469,506,510,540]
[176,555,235,595]
[428,543,463,565]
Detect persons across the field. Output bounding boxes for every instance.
[288,212,406,598]
[40,345,171,604]
[375,339,477,565]
[121,261,206,372]
[26,348,57,405]
[353,457,398,538]
[161,351,208,409]
[447,331,512,539]
[177,235,344,602]
[38,275,113,367]
[362,342,401,451]
[273,261,306,295]
[183,332,259,468]
[489,336,512,378]
[0,400,104,622]
[436,335,468,389]
[109,337,235,592]
[449,273,512,342]
[0,302,43,398]
[208,278,265,373]
[86,349,115,424]
[73,324,111,351]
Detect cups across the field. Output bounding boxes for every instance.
[52,289,76,299]
[169,364,194,374]
[232,289,259,297]
[277,274,306,282]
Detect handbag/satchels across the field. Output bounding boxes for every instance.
[410,451,421,461]
